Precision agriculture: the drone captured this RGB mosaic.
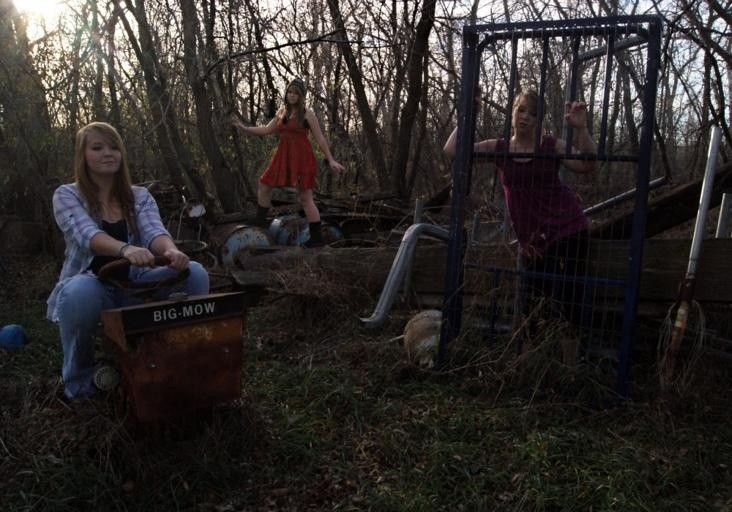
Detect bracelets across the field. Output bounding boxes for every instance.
[116,243,132,255]
[244,125,250,133]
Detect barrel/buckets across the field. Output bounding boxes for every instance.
[267,211,346,250]
[206,220,276,285]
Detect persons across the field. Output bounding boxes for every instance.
[230,79,345,247]
[442,86,597,357]
[45,122,210,400]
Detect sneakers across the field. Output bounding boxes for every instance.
[70,393,97,417]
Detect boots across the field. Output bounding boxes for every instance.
[240,206,270,228]
[300,222,325,248]
